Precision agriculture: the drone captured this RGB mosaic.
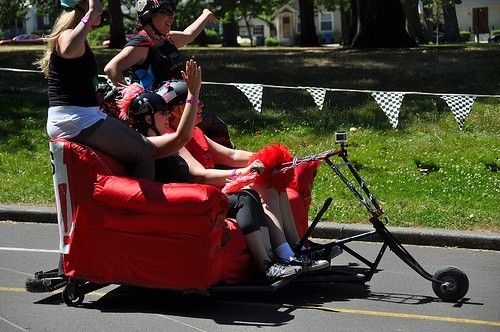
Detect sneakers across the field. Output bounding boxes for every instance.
[306,246,343,262]
[288,254,330,271]
[260,260,305,285]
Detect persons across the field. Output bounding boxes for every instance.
[33,0,190,183]
[117,83,302,277]
[103,0,233,150]
[157,79,342,271]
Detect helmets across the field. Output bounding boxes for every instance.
[159,78,188,109]
[115,82,168,121]
[60,0,84,8]
[136,0,180,19]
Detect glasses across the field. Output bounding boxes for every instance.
[160,8,175,17]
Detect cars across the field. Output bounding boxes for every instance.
[0,34,44,46]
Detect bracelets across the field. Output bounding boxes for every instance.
[186,99,199,104]
[232,169,236,180]
[81,16,91,26]
